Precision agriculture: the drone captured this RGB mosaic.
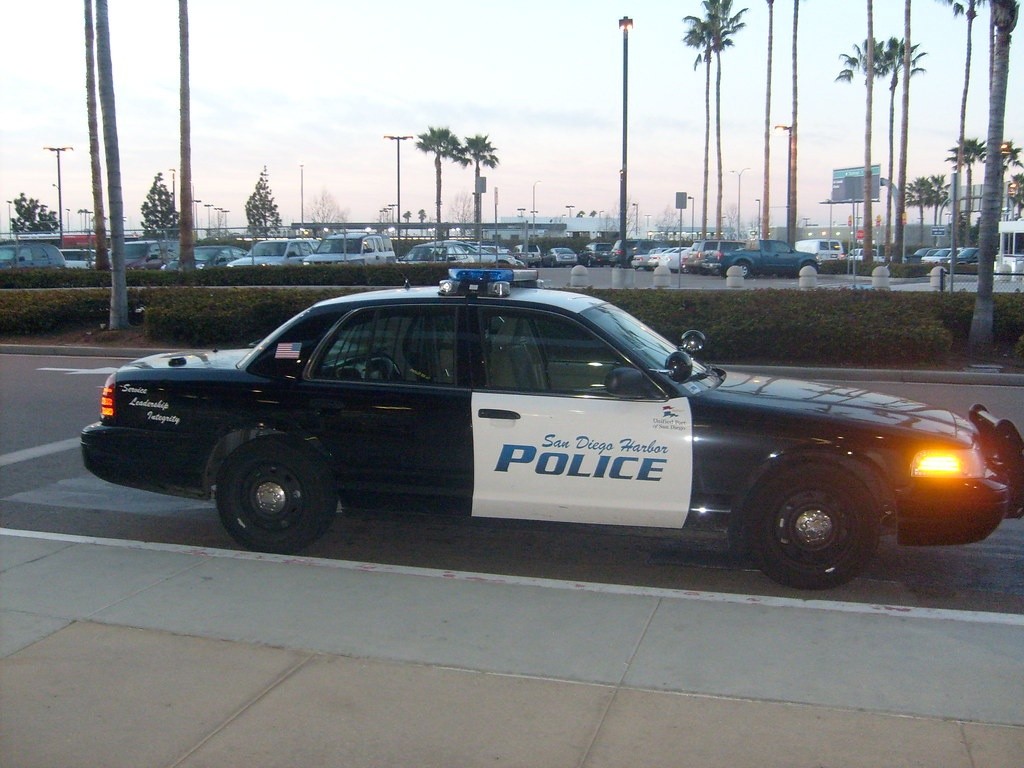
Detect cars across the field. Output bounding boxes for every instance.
[0,231,517,270]
[541,248,579,268]
[608,236,981,280]
[74,268,1024,596]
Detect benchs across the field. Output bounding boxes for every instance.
[320,350,412,379]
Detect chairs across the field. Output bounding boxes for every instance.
[512,317,549,391]
[490,315,521,393]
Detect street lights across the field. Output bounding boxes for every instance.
[383,134,415,260]
[773,124,793,242]
[213,208,223,236]
[6,200,12,238]
[85,211,95,265]
[730,168,751,240]
[299,164,306,238]
[565,205,575,217]
[42,145,74,249]
[632,203,638,239]
[530,180,543,245]
[168,168,176,241]
[193,199,202,242]
[204,204,215,229]
[1004,182,1018,222]
[616,13,635,268]
[756,198,761,239]
[517,208,526,222]
[222,210,231,236]
[688,196,695,243]
[65,208,70,234]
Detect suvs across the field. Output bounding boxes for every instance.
[511,243,542,268]
[576,241,614,268]
